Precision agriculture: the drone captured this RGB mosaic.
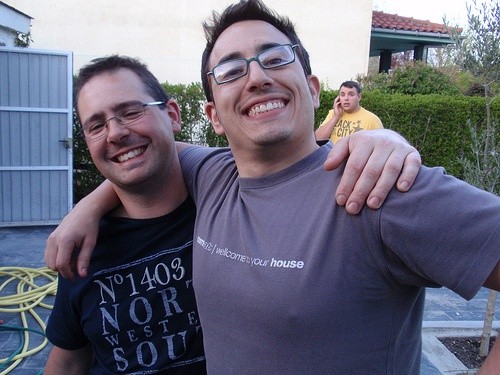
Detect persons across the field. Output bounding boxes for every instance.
[44,53,206,375]
[315,80,384,145]
[44,0,500,375]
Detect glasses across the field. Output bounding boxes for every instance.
[80,101,166,140]
[206,44,305,85]
